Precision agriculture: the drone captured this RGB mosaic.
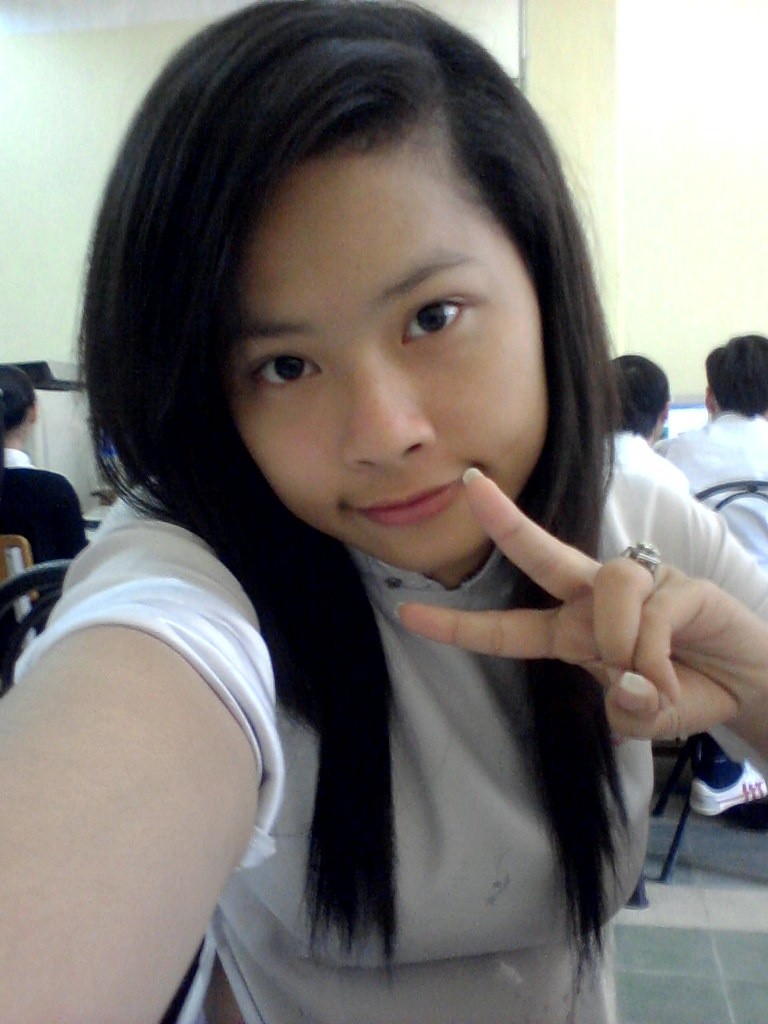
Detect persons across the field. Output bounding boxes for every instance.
[653,335,768,569]
[0,0,768,1024]
[611,355,768,815]
[0,363,87,565]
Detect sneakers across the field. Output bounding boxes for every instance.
[690,757,768,817]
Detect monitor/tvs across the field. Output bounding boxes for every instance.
[654,405,711,443]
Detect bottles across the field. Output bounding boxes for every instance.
[88,415,120,489]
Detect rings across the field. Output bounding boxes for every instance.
[621,541,661,583]
